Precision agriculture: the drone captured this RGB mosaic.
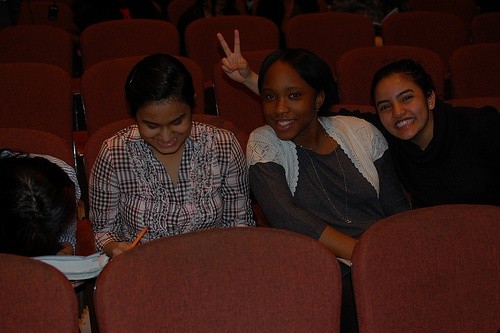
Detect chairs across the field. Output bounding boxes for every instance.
[0,0,500,333]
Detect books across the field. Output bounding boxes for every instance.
[31,251,110,282]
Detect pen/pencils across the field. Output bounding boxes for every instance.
[127,228,147,249]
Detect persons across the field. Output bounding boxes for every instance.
[245,47,411,276]
[88,53,256,259]
[0,148,85,289]
[216,29,500,211]
[0,0,500,47]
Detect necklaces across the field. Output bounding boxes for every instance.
[293,127,352,225]
